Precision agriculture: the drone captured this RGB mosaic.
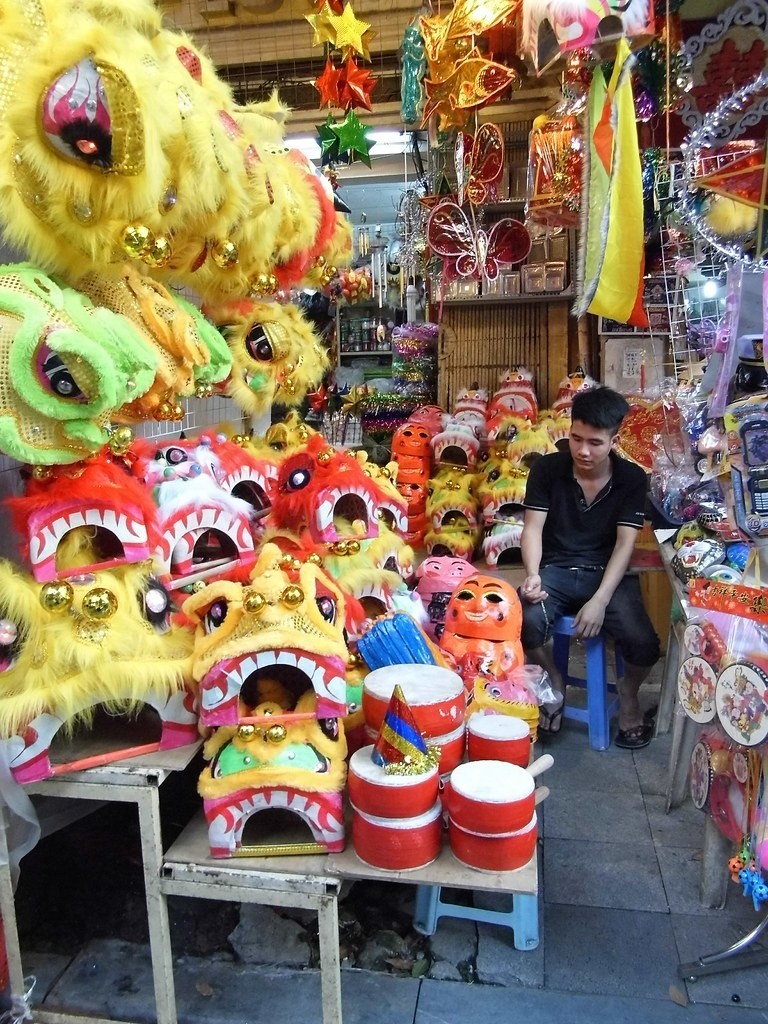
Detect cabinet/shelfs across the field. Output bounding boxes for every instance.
[597,227,693,335]
[336,298,410,383]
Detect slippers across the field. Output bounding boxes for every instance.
[538,671,565,739]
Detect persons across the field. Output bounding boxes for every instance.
[515,385,660,749]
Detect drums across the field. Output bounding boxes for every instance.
[447,758,537,834]
[447,810,537,875]
[352,795,443,873]
[362,662,467,816]
[468,716,532,769]
[347,742,440,822]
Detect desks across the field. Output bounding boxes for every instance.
[657,540,711,628]
[1,737,359,1024]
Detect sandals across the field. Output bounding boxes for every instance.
[615,711,653,749]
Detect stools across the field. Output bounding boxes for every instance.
[651,608,733,913]
[413,879,539,949]
[550,615,623,751]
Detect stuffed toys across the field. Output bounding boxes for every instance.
[1,0,658,867]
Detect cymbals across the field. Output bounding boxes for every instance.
[708,768,747,843]
